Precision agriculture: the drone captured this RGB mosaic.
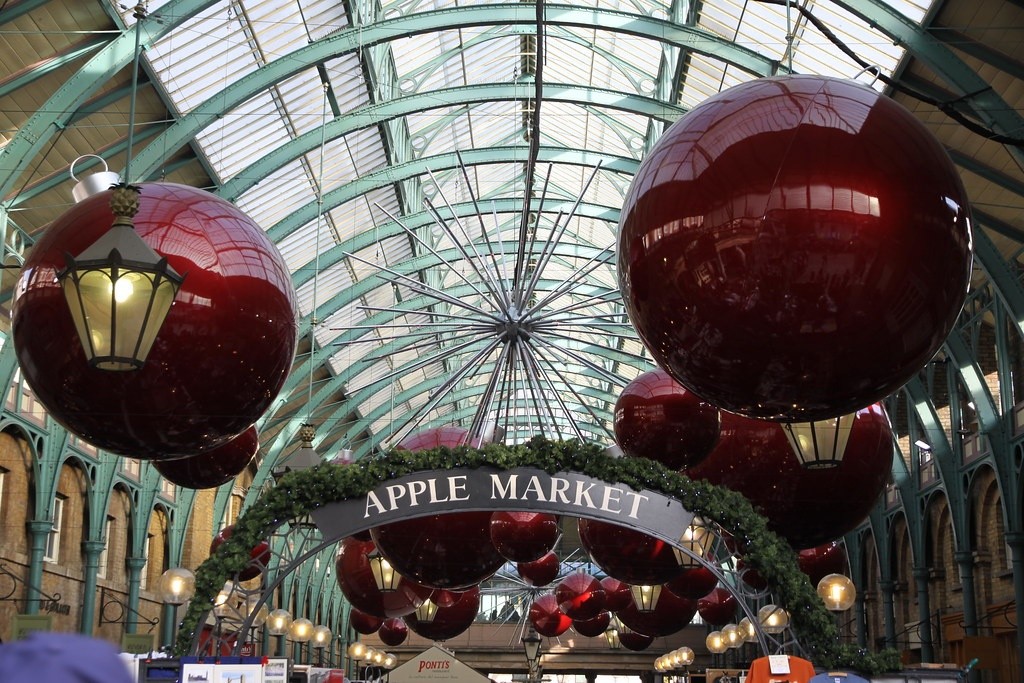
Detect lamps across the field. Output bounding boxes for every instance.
[266,609,293,657]
[382,653,397,683]
[362,645,377,681]
[348,642,368,681]
[654,646,694,683]
[290,618,315,664]
[672,513,716,569]
[371,649,387,682]
[520,624,542,662]
[780,411,856,469]
[629,584,662,613]
[270,423,323,530]
[416,597,439,622]
[51,1,189,372]
[310,624,333,667]
[759,604,787,655]
[366,547,402,592]
[238,598,268,656]
[605,612,623,650]
[159,567,197,651]
[706,630,729,668]
[214,585,238,658]
[720,623,744,668]
[914,437,931,452]
[816,573,856,642]
[739,616,759,660]
